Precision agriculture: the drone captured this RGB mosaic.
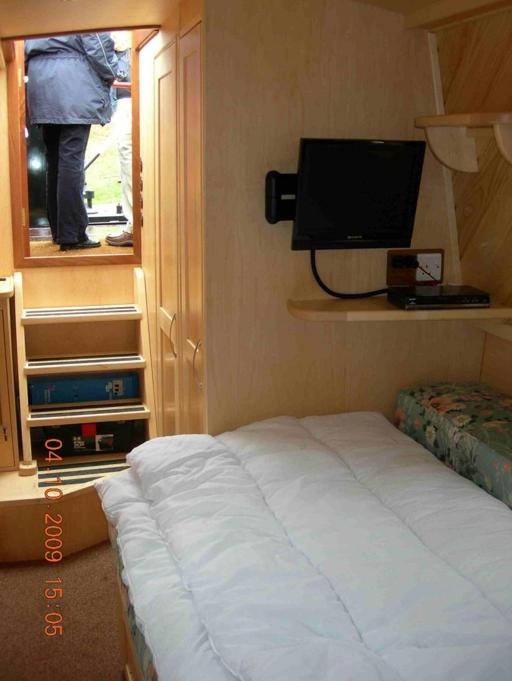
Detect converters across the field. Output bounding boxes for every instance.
[392,257,419,268]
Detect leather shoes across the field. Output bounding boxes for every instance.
[52,237,102,249]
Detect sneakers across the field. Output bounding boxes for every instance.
[105,230,133,246]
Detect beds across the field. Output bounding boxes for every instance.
[93,408,511,680]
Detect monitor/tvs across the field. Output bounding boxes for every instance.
[265,138,427,251]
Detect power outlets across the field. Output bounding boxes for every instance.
[387,249,444,284]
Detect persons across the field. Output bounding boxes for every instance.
[106,29,133,246]
[24,31,118,251]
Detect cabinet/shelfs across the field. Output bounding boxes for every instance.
[287,111,512,322]
